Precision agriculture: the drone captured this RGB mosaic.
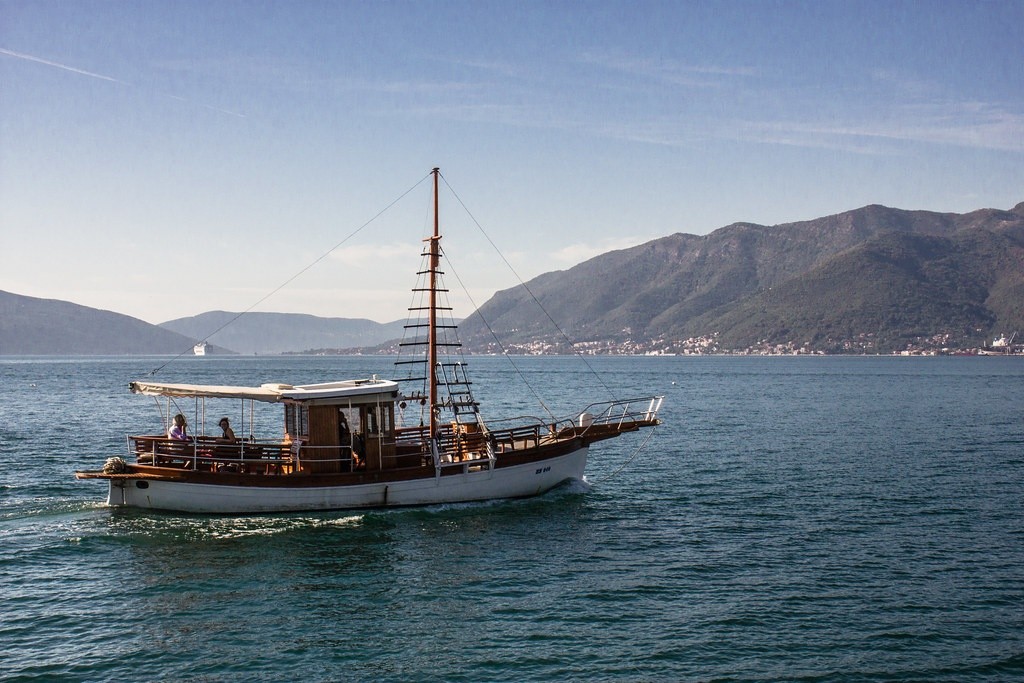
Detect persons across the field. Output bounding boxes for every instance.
[168,414,193,468]
[339,411,365,468]
[212,417,238,472]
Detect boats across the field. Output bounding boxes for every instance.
[73,167,667,519]
[192,341,214,356]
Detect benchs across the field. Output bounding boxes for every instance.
[420,424,540,466]
[129,435,297,466]
